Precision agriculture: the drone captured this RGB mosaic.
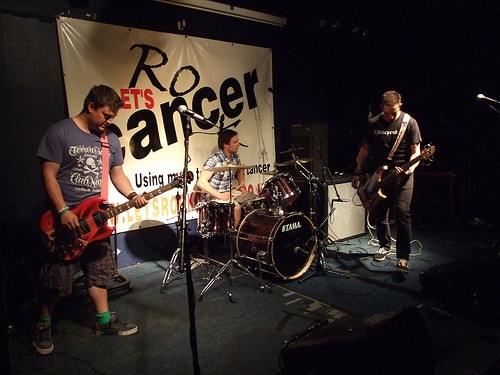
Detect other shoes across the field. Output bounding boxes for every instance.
[397,258,410,272]
[373,248,390,261]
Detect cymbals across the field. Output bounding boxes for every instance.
[279,147,307,154]
[275,157,315,166]
[262,170,284,175]
[203,164,257,172]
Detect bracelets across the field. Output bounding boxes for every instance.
[126,192,137,200]
[58,206,69,215]
[353,173,361,176]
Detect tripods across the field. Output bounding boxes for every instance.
[158,116,351,303]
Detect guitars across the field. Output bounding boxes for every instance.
[40,171,194,261]
[357,143,435,213]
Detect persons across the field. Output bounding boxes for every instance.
[196,129,252,229]
[351,91,422,272]
[29,84,148,354]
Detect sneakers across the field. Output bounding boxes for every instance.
[94,310,138,336]
[31,321,54,354]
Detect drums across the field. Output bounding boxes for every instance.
[259,173,301,209]
[232,207,318,279]
[204,200,234,232]
[240,197,268,216]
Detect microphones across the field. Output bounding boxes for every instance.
[179,105,215,126]
[477,93,500,104]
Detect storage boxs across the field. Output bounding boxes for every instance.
[294,173,367,245]
